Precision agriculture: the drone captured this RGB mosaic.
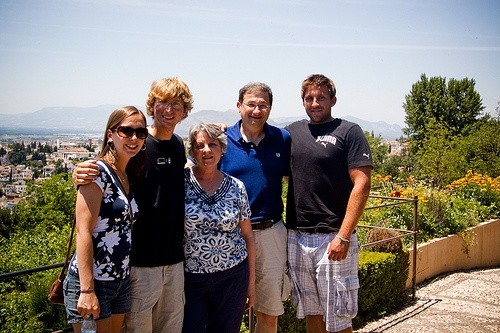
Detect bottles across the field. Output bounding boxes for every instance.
[80,314,97,333]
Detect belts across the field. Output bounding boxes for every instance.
[251,220,274,231]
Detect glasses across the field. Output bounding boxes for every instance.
[155,99,184,110]
[241,101,270,109]
[111,126,148,139]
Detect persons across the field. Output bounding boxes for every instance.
[283,74,373,333]
[73,77,193,333]
[62,107,149,333]
[185,82,291,333]
[183,123,255,333]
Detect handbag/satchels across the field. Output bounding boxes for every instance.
[48,274,66,304]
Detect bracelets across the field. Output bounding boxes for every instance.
[80,290,95,294]
[336,235,350,243]
